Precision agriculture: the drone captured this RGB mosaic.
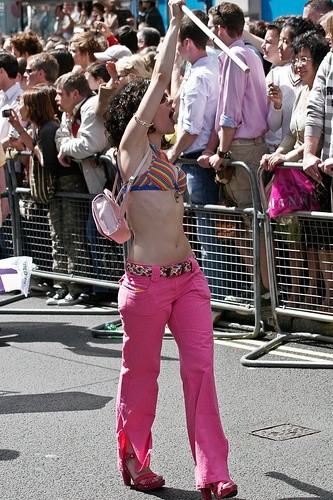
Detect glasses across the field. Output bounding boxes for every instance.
[160,93,168,103]
[215,5,226,23]
[117,75,126,79]
[293,56,312,64]
[25,68,36,74]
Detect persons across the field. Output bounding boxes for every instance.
[106,0,240,500]
[0,0,333,312]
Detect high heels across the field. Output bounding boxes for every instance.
[121,453,165,491]
[201,478,237,500]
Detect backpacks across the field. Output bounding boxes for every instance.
[92,147,150,244]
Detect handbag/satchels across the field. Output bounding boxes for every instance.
[216,201,245,239]
[27,156,56,204]
[268,164,321,218]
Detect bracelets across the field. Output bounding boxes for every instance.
[0,191,10,199]
[215,147,233,159]
[132,113,154,128]
[18,130,25,135]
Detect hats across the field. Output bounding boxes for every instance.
[93,44,132,62]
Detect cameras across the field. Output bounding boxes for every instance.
[2,110,11,117]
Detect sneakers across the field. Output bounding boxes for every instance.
[46,289,68,304]
[237,289,273,311]
[226,289,253,303]
[58,292,86,306]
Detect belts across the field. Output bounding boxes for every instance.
[230,137,266,146]
[126,256,197,278]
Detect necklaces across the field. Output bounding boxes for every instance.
[150,143,159,152]
